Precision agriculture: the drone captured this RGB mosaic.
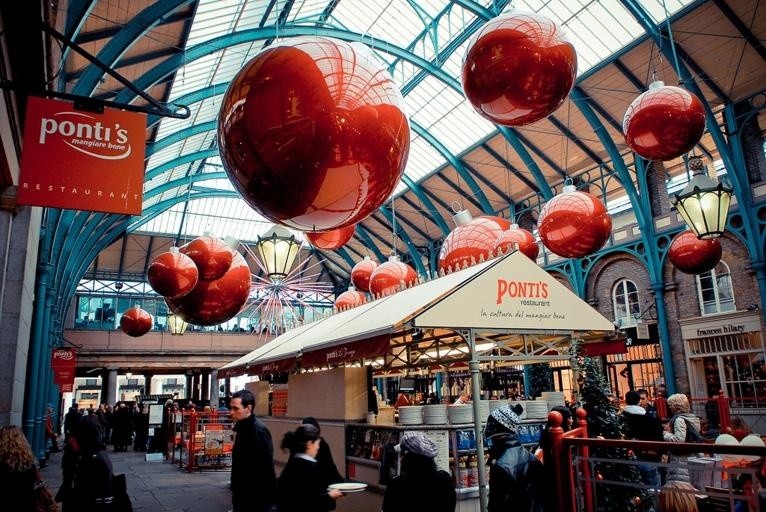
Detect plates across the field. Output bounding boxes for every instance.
[398,391,572,426]
[326,480,369,494]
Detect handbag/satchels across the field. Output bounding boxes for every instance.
[30,462,59,512]
[667,413,708,456]
[60,474,132,512]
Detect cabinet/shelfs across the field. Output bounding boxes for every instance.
[340,416,548,505]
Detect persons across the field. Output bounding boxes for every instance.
[302,417,349,511]
[276,424,346,511]
[115,399,229,457]
[229,389,278,511]
[53,402,114,511]
[1,425,58,510]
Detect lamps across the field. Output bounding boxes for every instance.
[669,150,736,242]
[439,375,502,399]
[253,223,306,282]
[163,309,191,336]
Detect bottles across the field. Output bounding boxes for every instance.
[455,452,492,498]
[367,408,376,424]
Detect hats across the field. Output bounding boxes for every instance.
[392,430,440,458]
[490,399,525,435]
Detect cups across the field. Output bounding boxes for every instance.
[706,453,721,461]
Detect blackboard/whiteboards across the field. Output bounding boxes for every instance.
[148,404,164,425]
